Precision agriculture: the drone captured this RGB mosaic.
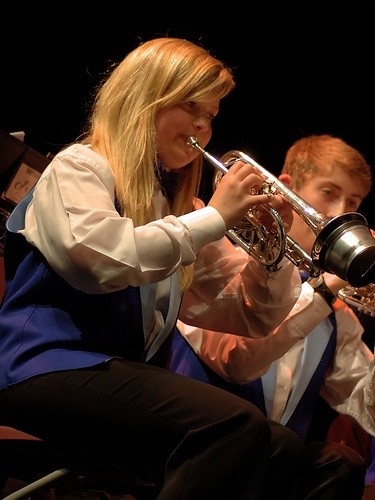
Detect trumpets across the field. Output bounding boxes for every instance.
[332,280,374,315]
[182,135,375,294]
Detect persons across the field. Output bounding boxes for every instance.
[167,133,374,500]
[0,36,321,500]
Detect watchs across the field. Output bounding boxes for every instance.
[307,273,337,307]
